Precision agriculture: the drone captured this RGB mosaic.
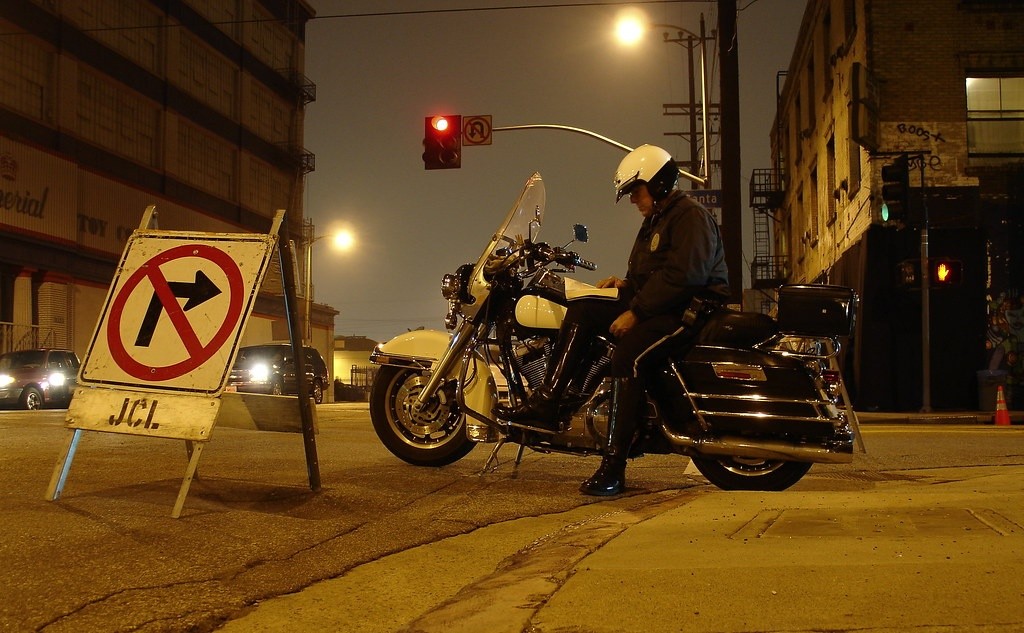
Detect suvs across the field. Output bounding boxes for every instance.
[225,342,330,404]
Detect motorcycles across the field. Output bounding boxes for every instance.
[369,172,866,491]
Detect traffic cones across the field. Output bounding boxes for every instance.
[992,385,1011,426]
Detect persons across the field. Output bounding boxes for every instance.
[491,142,730,498]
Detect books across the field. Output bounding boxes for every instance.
[564,276,620,302]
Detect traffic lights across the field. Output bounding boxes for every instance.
[422,114,462,170]
[880,152,911,223]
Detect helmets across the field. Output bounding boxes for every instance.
[612,143,680,204]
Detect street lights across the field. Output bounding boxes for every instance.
[616,12,710,183]
[302,232,353,350]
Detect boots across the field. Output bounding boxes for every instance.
[578,375,649,495]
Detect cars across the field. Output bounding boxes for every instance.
[0,349,80,410]
